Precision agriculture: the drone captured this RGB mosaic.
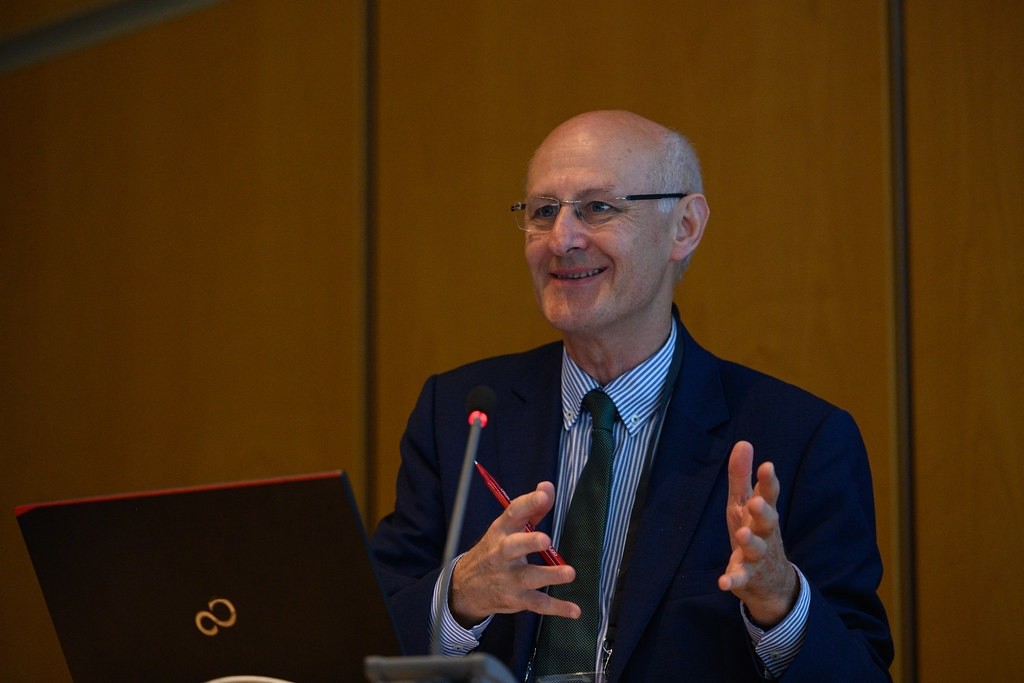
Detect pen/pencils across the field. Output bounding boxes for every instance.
[474,460,566,567]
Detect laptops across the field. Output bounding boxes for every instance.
[14,470,405,683]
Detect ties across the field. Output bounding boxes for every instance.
[531,389,618,683]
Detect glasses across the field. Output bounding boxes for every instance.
[511,193,687,234]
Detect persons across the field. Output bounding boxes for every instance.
[372,109,899,683]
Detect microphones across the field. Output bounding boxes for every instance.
[364,385,519,683]
